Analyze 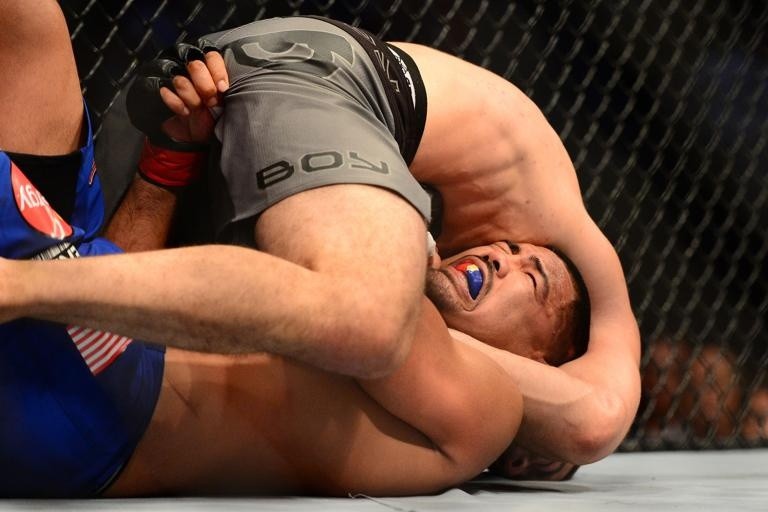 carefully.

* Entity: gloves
[127,36,226,151]
